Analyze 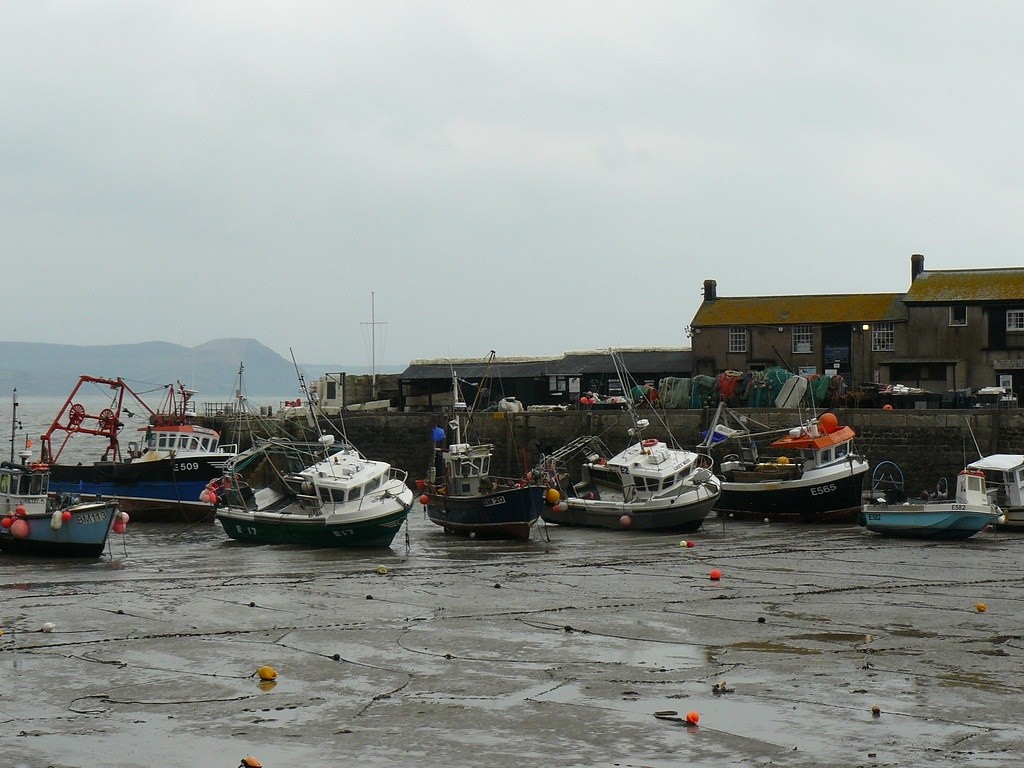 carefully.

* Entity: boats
[695,362,870,525]
[965,415,1024,531]
[27,375,238,525]
[198,345,415,547]
[531,346,727,535]
[0,388,122,559]
[415,349,549,542]
[860,461,1008,541]
